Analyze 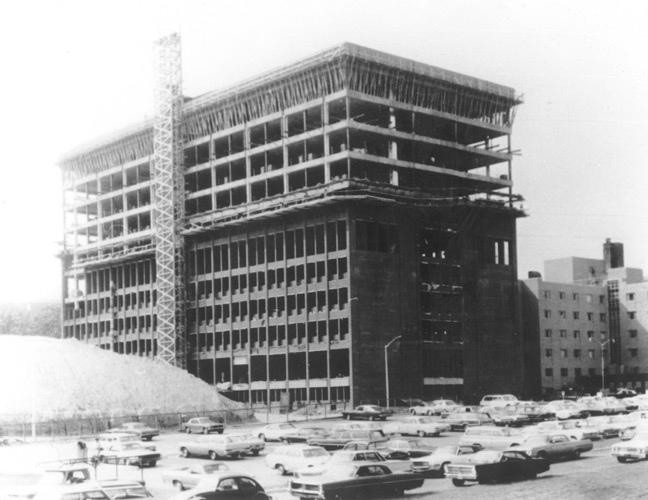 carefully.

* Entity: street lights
[384,335,405,408]
[588,336,617,390]
